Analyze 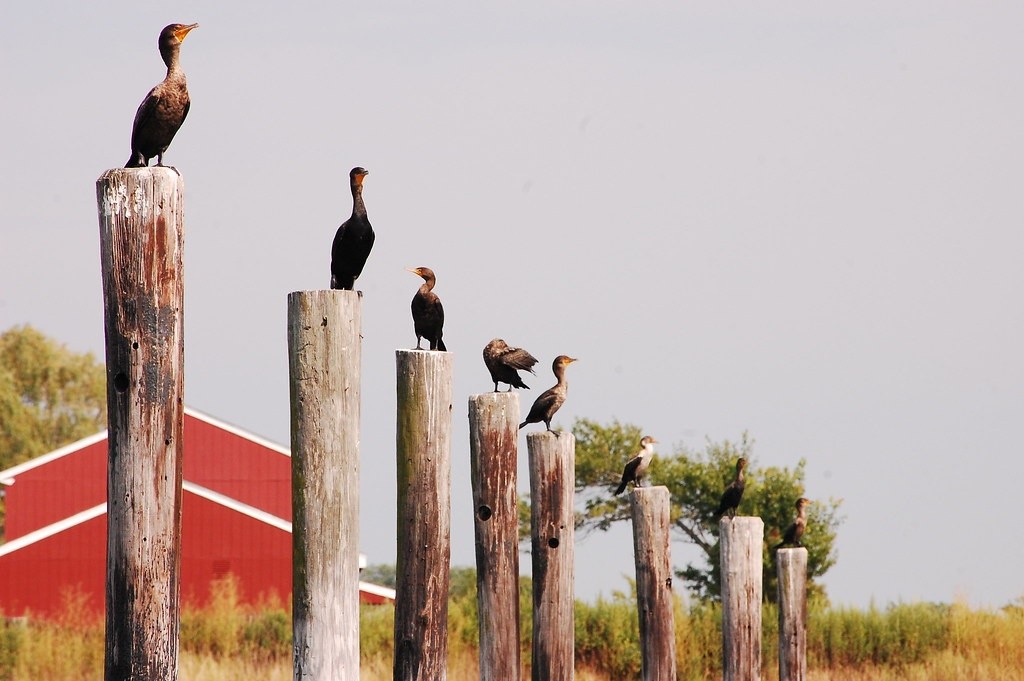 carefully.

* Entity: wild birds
[405,267,449,352]
[328,165,376,298]
[482,338,539,392]
[615,436,659,496]
[717,458,748,517]
[518,355,578,438]
[123,20,200,177]
[773,498,814,549]
